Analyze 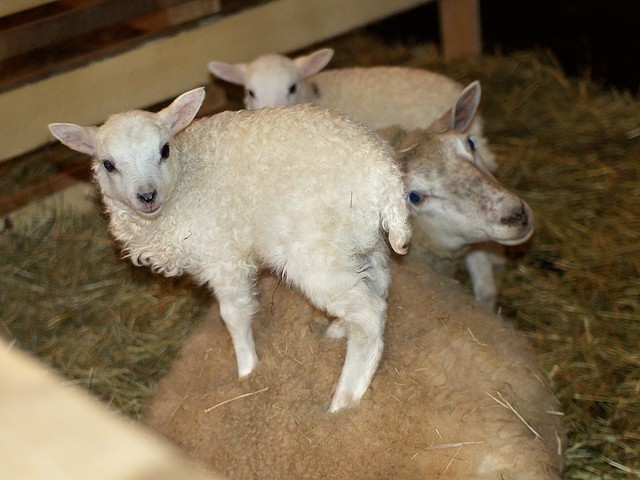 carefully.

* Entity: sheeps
[208,50,497,309]
[47,85,411,414]
[139,80,563,480]
